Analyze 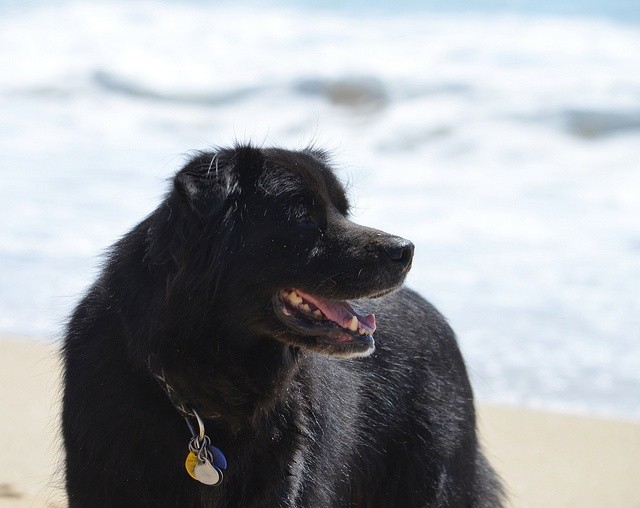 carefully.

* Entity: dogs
[56,138,512,508]
